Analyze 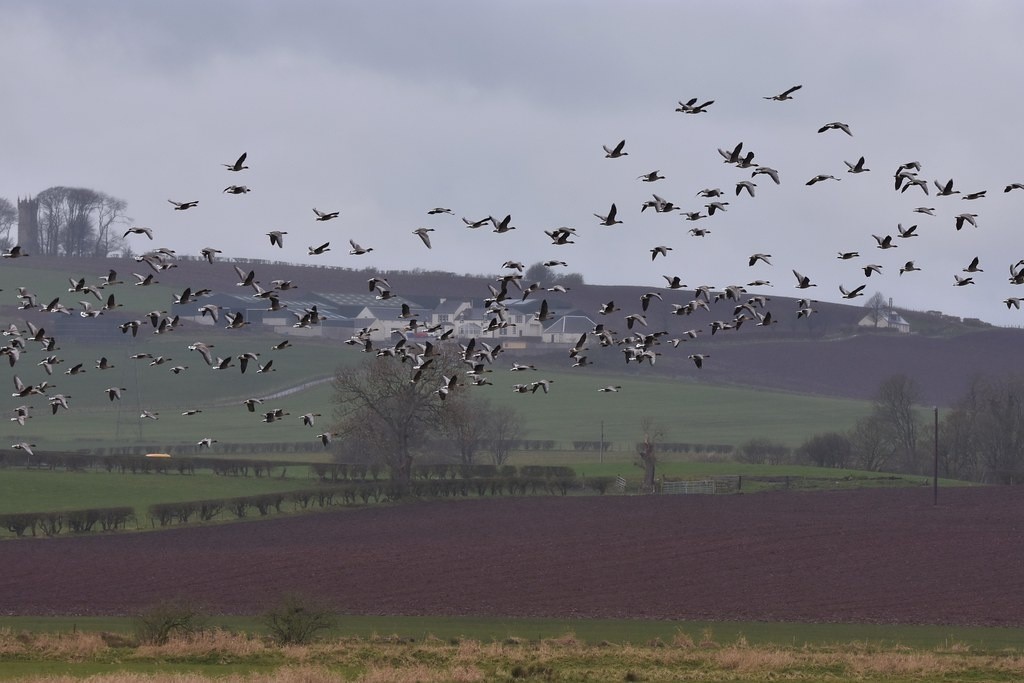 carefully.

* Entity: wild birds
[899,261,921,277]
[836,252,860,259]
[963,256,984,272]
[602,139,629,158]
[544,229,575,244]
[952,275,975,286]
[818,122,853,137]
[793,270,818,289]
[762,84,803,101]
[913,207,935,215]
[641,193,681,213]
[462,216,491,228]
[796,298,818,308]
[220,152,249,172]
[695,188,725,197]
[428,207,455,215]
[954,213,979,230]
[312,207,340,221]
[726,150,759,168]
[717,142,745,163]
[637,170,665,182]
[552,227,581,238]
[316,432,334,447]
[872,234,898,249]
[222,185,251,194]
[704,202,729,216]
[894,172,917,190]
[489,214,516,233]
[593,203,623,226]
[897,223,919,238]
[862,264,883,277]
[675,98,697,112]
[687,227,712,237]
[751,167,781,186]
[0,239,780,427]
[839,284,866,299]
[679,210,707,220]
[893,161,922,177]
[685,100,715,114]
[198,438,217,448]
[961,190,987,200]
[736,181,757,197]
[412,228,435,249]
[265,231,288,248]
[168,200,199,210]
[1003,297,1024,309]
[934,178,961,196]
[123,226,154,240]
[11,442,37,455]
[1009,260,1024,285]
[844,156,870,173]
[805,174,842,185]
[1004,183,1024,193]
[796,309,818,319]
[901,180,929,196]
